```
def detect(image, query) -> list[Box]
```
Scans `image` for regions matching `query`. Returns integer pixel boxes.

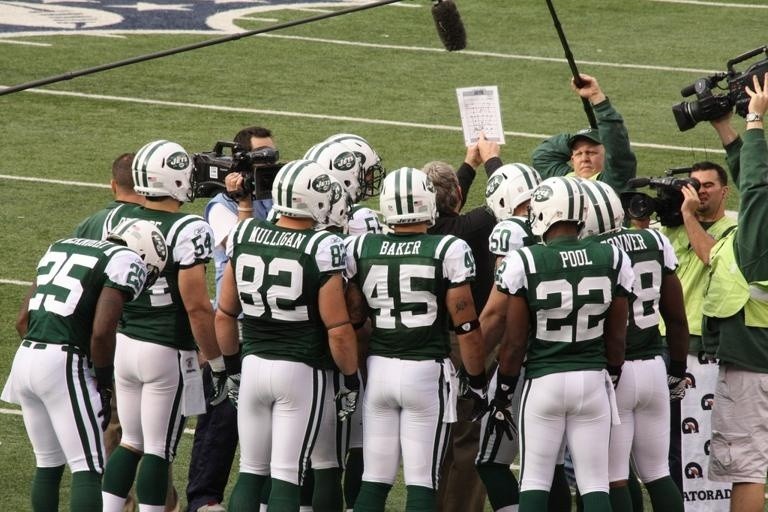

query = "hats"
[568,128,601,146]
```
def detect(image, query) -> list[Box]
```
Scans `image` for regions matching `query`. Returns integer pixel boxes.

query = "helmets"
[106,218,168,289]
[486,163,625,238]
[269,134,437,230]
[132,140,193,201]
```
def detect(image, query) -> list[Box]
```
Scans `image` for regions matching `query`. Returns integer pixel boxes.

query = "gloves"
[98,385,112,431]
[465,370,518,440]
[210,371,241,409]
[336,374,360,421]
[668,361,687,401]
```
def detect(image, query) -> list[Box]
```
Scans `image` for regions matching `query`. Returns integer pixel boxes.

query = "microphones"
[432,0,466,52]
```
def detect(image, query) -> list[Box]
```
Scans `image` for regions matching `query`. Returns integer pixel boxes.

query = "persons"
[0,72,768,512]
